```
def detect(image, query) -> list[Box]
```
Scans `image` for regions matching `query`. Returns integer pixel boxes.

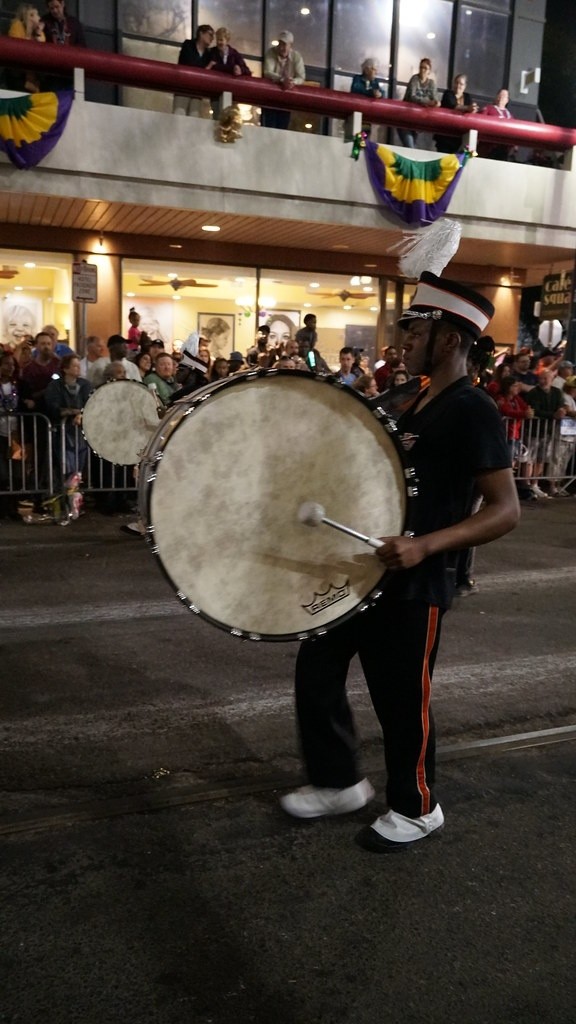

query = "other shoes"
[454,580,479,598]
[121,522,142,536]
[279,778,376,818]
[531,485,548,498]
[356,802,445,852]
[550,485,570,497]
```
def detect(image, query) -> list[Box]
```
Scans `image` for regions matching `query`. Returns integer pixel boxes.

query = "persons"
[174,25,251,120]
[467,335,576,500]
[264,314,298,347]
[433,74,479,153]
[284,220,521,842]
[0,310,332,533]
[336,345,408,399]
[0,0,85,93]
[200,317,231,360]
[397,59,440,148]
[261,31,305,129]
[351,60,385,140]
[477,89,514,161]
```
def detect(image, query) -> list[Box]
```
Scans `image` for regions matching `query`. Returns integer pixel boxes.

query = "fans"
[139,279,218,290]
[311,288,377,300]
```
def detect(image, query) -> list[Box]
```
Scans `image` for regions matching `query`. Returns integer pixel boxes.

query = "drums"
[138,366,422,642]
[78,380,167,469]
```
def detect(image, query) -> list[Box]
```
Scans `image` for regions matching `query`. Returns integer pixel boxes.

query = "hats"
[559,360,576,368]
[108,335,132,347]
[227,352,244,365]
[353,346,364,353]
[151,339,164,348]
[258,325,270,334]
[175,349,209,375]
[385,217,495,339]
